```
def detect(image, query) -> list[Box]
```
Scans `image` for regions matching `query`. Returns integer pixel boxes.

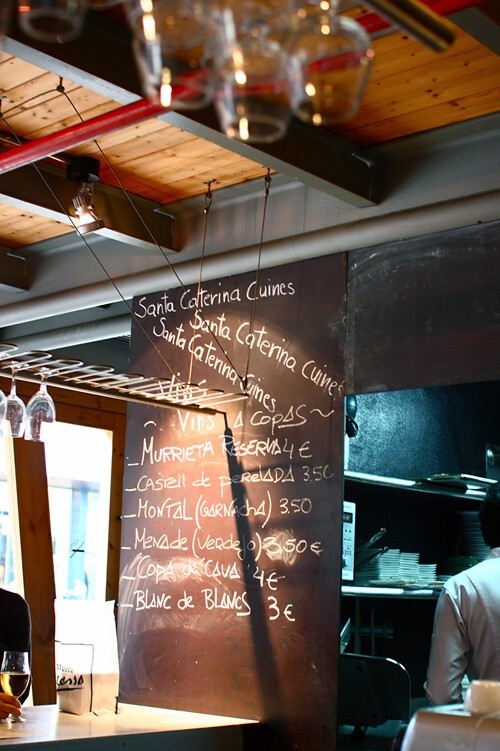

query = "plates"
[354,548,438,587]
[408,474,498,491]
[455,511,491,557]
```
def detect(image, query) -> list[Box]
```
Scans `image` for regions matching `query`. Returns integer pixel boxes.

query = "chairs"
[338,653,411,751]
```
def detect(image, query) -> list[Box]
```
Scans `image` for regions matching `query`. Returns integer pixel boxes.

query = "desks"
[0,702,259,751]
[401,703,500,751]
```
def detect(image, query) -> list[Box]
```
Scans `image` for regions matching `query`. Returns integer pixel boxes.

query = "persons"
[0,588,31,723]
[423,480,500,708]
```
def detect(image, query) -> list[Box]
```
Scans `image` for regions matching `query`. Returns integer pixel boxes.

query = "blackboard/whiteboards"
[119,252,346,751]
[345,220,500,397]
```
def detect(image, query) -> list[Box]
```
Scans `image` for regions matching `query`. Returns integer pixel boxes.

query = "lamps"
[66,156,105,236]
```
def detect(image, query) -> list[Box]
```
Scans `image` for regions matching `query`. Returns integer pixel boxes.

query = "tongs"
[354,527,389,573]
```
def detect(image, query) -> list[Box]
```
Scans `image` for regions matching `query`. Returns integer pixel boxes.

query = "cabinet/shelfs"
[340,470,484,652]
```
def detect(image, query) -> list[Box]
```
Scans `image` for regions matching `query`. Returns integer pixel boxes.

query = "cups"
[465,679,500,713]
[17,0,371,140]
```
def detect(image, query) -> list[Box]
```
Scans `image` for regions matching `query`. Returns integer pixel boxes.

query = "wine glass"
[0,650,31,724]
